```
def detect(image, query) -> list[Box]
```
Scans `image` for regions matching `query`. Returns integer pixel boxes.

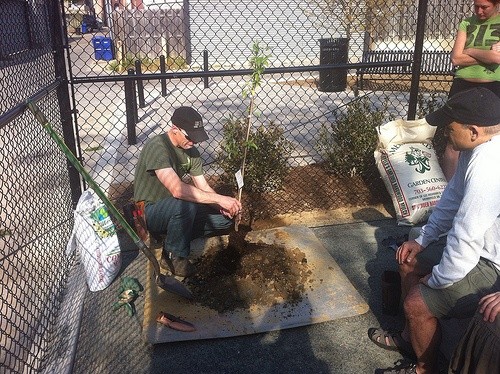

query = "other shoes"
[160,249,193,277]
[156,233,166,245]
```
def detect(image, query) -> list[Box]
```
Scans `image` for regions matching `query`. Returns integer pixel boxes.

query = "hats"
[171,106,209,144]
[425,87,500,127]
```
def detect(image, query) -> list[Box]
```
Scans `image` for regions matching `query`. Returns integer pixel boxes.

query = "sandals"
[376,363,417,374]
[368,328,415,352]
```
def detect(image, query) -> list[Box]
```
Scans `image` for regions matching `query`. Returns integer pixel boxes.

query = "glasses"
[179,128,191,140]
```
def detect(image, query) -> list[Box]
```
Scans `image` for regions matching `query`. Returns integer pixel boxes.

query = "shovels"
[24,98,195,302]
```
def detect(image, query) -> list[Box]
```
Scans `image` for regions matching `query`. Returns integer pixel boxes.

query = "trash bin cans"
[319,38,349,93]
[75,28,81,35]
[82,23,87,34]
[92,36,114,62]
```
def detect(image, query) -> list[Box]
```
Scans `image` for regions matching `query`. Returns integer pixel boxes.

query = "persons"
[446,290,500,374]
[369,88,499,374]
[133,107,242,277]
[440,0,500,181]
